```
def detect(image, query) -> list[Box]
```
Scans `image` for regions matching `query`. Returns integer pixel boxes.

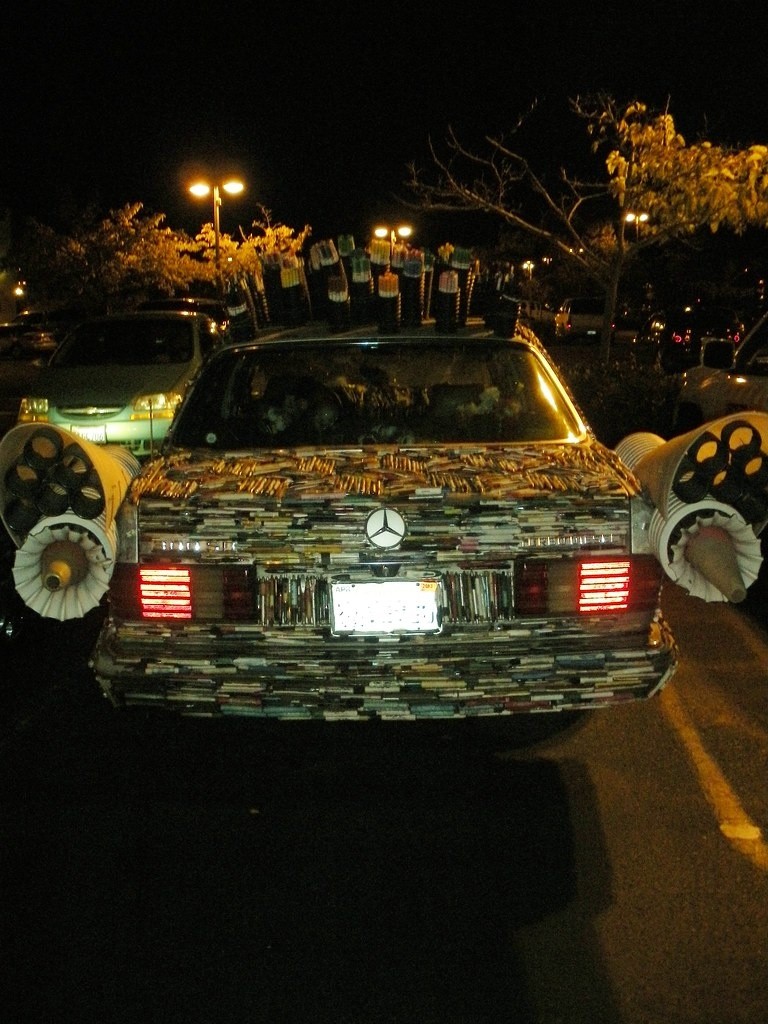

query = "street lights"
[374,226,413,270]
[522,261,535,281]
[189,176,244,298]
[627,213,649,240]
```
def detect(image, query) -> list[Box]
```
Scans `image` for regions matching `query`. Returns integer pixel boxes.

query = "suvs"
[554,295,614,344]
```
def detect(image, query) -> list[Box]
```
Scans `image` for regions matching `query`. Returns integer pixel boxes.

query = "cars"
[0,236,768,744]
[133,299,254,343]
[669,310,768,441]
[611,317,641,345]
[0,310,47,358]
[19,310,226,458]
[638,309,746,373]
[21,311,85,352]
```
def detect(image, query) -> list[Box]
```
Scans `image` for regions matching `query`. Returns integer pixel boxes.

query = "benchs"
[243,388,494,445]
